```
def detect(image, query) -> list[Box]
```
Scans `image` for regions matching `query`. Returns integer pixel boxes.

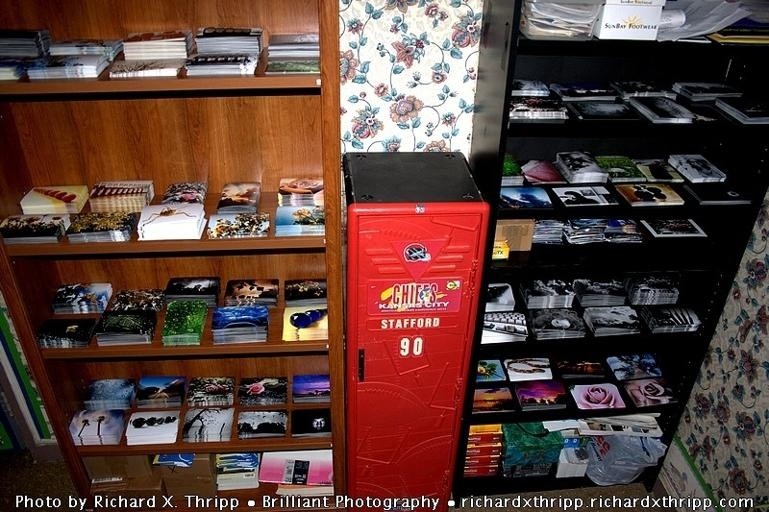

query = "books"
[0,178,325,243]
[215,450,334,497]
[498,154,752,208]
[508,79,769,125]
[480,274,701,344]
[578,417,664,437]
[69,376,331,446]
[464,423,503,477]
[519,0,769,42]
[0,27,320,80]
[36,276,328,348]
[532,217,709,243]
[472,355,678,414]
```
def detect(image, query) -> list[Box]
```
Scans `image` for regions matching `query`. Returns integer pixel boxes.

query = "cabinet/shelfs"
[1,0,347,512]
[451,0,769,510]
